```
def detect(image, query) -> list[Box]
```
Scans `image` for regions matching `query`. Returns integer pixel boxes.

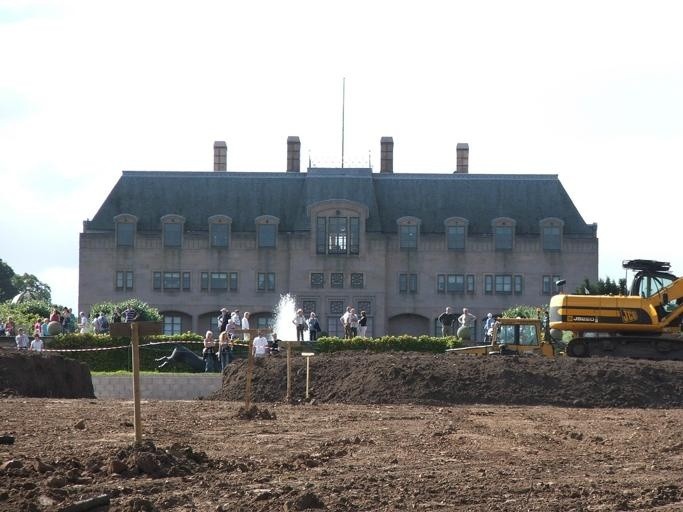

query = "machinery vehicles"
[443,317,566,362]
[548,275,682,361]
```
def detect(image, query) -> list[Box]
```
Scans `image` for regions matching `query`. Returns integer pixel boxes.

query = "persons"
[204,308,281,373]
[0,302,139,351]
[438,306,456,337]
[340,305,368,338]
[292,308,305,341]
[458,308,477,327]
[483,312,493,342]
[308,312,318,341]
